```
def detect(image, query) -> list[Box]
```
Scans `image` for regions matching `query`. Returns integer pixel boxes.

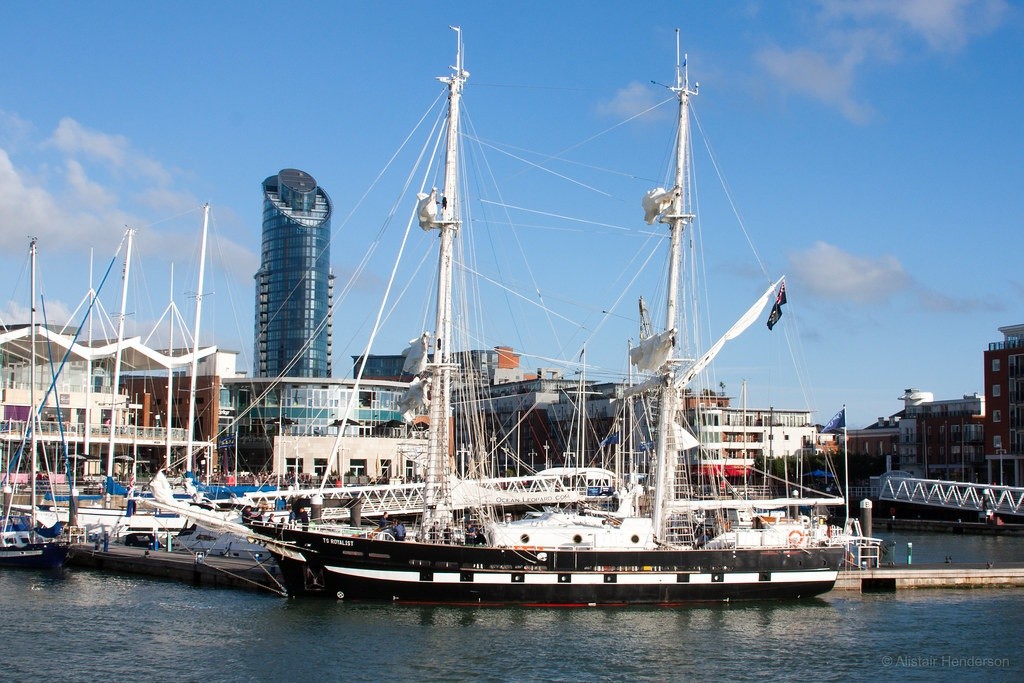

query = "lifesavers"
[788,530,803,546]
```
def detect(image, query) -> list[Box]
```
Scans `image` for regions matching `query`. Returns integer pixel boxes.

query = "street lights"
[528,449,537,469]
[456,442,470,479]
[562,445,575,467]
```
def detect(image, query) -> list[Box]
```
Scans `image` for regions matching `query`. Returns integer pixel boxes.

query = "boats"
[868,471,1024,533]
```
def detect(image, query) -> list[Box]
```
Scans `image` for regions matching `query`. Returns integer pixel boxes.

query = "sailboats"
[0,25,884,608]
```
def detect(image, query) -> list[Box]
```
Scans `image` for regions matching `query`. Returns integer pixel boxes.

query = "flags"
[766,281,788,331]
[818,409,845,433]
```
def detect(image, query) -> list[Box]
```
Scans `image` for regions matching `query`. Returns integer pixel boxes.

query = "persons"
[277,517,289,530]
[288,509,296,529]
[44,473,50,486]
[428,521,443,544]
[392,520,406,541]
[406,474,424,483]
[35,471,43,485]
[265,513,276,528]
[371,476,389,484]
[256,511,266,526]
[47,414,54,421]
[316,471,342,489]
[464,522,477,546]
[242,505,253,525]
[379,512,392,540]
[474,529,487,546]
[292,507,309,532]
[200,471,313,489]
[443,523,454,545]
